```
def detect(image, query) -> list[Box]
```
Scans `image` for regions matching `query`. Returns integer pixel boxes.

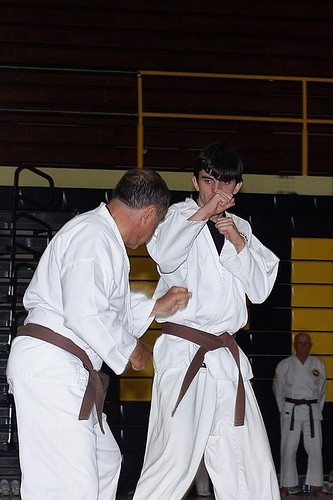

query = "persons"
[6,169,193,500]
[131,146,282,500]
[273,333,328,498]
[196,454,214,500]
